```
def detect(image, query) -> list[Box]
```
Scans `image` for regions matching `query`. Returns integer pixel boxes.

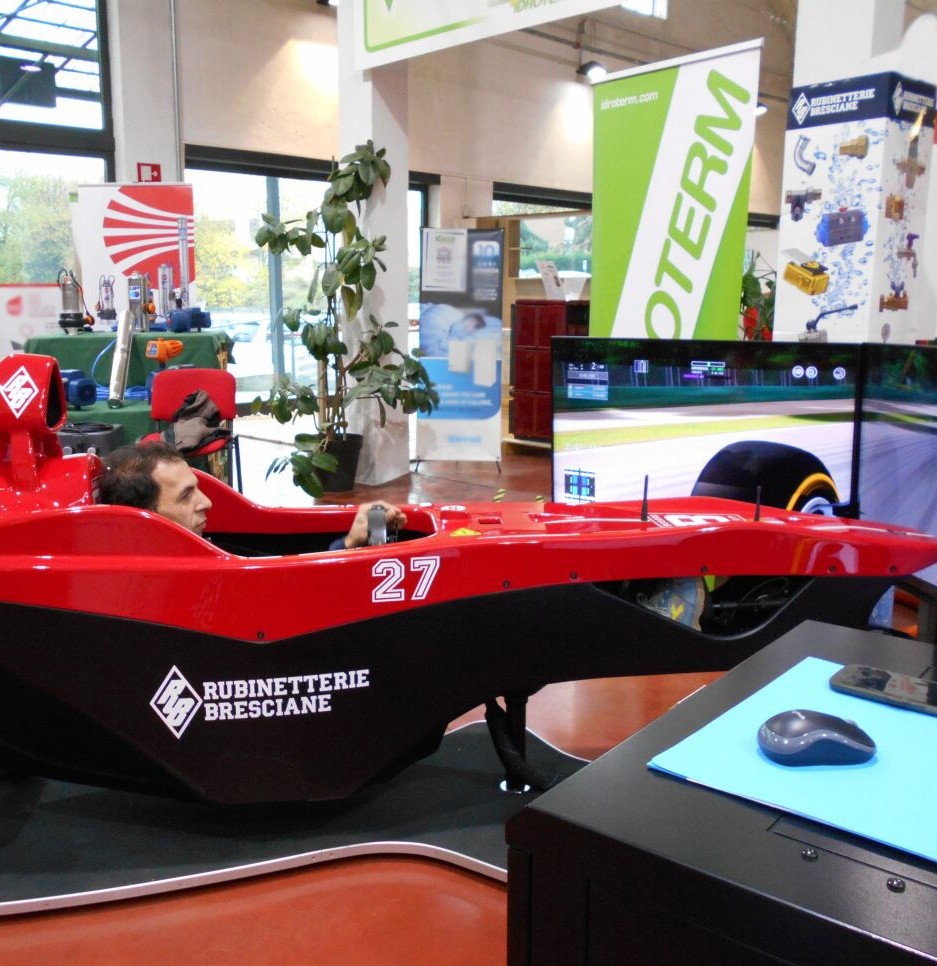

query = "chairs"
[150,367,243,494]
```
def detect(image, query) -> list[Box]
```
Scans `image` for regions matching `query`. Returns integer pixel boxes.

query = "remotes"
[831,664,936,714]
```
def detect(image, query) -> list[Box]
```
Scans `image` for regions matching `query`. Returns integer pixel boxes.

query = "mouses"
[759,710,877,764]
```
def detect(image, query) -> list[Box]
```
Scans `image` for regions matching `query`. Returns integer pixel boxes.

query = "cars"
[230,316,314,354]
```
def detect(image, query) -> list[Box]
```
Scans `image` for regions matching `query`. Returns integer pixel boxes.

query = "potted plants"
[255,140,440,497]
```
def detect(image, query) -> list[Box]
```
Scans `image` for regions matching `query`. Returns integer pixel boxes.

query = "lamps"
[577,60,605,79]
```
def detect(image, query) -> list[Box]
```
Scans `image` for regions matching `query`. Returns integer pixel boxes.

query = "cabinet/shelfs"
[507,299,590,438]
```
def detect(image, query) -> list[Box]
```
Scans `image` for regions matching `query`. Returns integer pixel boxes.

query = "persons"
[96,440,408,551]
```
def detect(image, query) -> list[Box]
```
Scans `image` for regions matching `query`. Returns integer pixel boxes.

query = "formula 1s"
[2,352,937,811]
